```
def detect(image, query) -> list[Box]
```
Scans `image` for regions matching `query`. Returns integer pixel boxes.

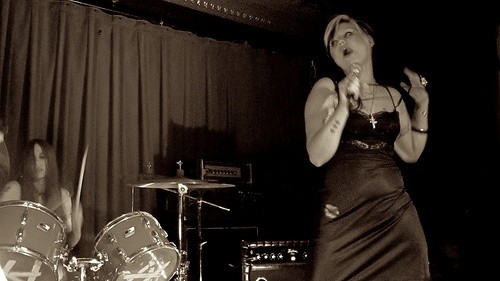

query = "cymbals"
[125,178,236,190]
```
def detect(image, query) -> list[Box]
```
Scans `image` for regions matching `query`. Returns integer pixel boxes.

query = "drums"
[0,200,70,281]
[67,256,96,280]
[90,210,182,281]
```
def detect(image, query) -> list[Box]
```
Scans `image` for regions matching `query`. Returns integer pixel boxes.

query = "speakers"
[157,189,316,281]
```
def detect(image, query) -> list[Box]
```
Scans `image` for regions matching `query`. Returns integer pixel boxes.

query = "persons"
[305,14,430,281]
[0,118,84,281]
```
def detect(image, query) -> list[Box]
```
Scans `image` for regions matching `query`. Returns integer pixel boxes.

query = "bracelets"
[411,126,429,134]
[414,108,428,118]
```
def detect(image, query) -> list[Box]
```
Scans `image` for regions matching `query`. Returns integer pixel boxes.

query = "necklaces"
[38,191,43,196]
[363,81,377,128]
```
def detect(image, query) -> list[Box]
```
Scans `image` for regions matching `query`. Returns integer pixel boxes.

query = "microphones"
[349,63,361,111]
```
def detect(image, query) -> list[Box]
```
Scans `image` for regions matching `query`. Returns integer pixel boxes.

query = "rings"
[419,75,428,86]
[407,86,411,94]
